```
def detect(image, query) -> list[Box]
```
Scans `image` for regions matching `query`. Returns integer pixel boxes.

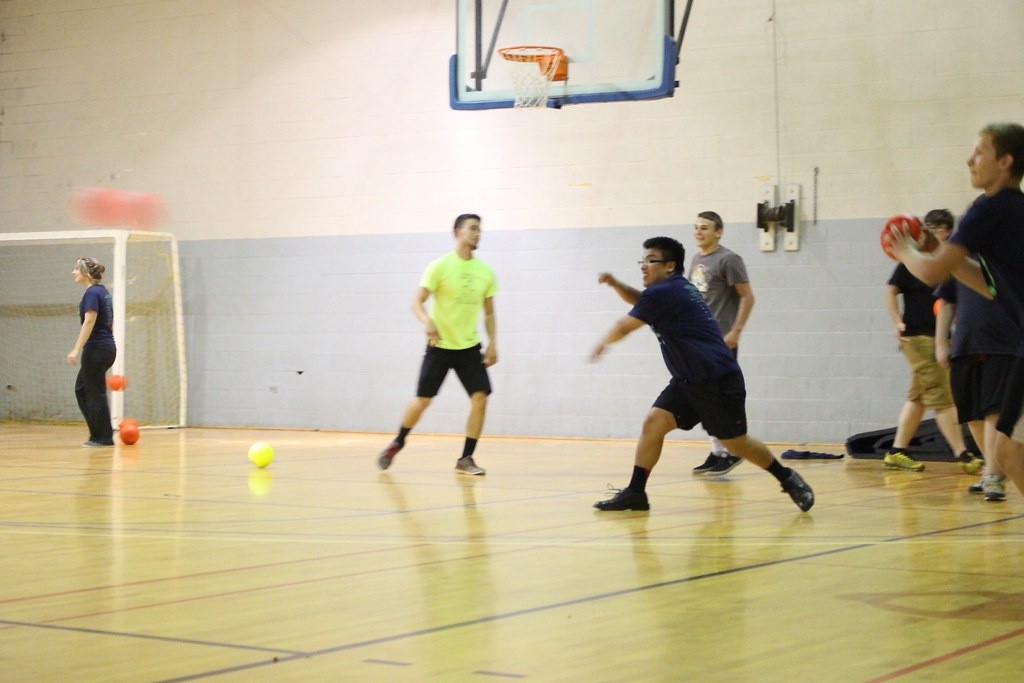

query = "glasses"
[638,260,675,267]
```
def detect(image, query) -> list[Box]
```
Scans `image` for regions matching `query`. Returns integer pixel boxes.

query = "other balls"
[880,215,931,263]
[119,418,139,430]
[248,442,274,468]
[120,425,140,445]
[106,374,122,391]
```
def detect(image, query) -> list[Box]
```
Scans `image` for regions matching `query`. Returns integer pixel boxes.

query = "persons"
[881,122,1024,500]
[685,210,754,477]
[589,237,816,513]
[378,213,499,477]
[68,258,117,447]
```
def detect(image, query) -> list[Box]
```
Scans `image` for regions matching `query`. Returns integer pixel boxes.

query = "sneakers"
[957,452,985,474]
[379,436,404,469]
[710,455,744,476]
[983,473,1007,500]
[881,450,925,472]
[456,454,485,476]
[692,451,723,473]
[969,479,984,494]
[593,484,649,510]
[780,467,814,511]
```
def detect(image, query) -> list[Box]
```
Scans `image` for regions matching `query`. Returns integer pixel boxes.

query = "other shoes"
[83,441,116,448]
